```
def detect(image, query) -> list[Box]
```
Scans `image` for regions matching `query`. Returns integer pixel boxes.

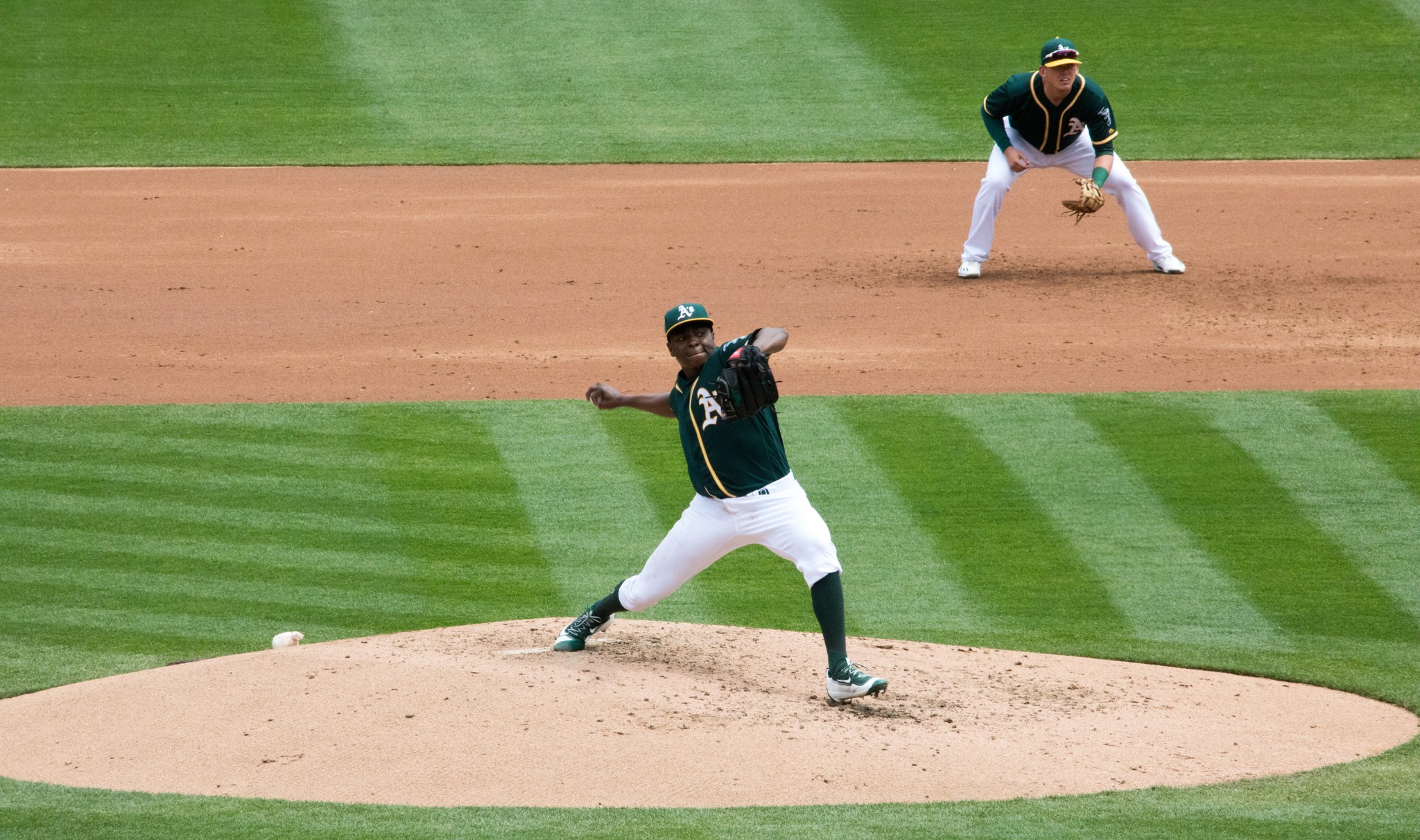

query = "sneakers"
[1153,254,1186,272]
[959,260,981,278]
[826,656,889,704]
[554,604,615,652]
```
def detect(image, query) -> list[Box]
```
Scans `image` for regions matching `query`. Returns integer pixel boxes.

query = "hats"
[1040,36,1083,67]
[664,303,714,339]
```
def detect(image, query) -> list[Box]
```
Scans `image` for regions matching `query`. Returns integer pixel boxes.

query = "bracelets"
[1092,167,1110,189]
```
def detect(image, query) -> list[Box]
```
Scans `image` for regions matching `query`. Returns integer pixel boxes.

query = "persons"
[554,304,888,703]
[957,36,1186,276]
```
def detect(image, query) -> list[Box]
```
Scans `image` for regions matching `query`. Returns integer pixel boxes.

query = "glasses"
[1042,50,1080,64]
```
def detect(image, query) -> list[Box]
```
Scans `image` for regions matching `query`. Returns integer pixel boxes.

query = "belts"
[759,488,768,494]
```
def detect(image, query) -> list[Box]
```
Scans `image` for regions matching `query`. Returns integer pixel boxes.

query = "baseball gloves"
[1062,178,1105,214]
[717,345,778,424]
